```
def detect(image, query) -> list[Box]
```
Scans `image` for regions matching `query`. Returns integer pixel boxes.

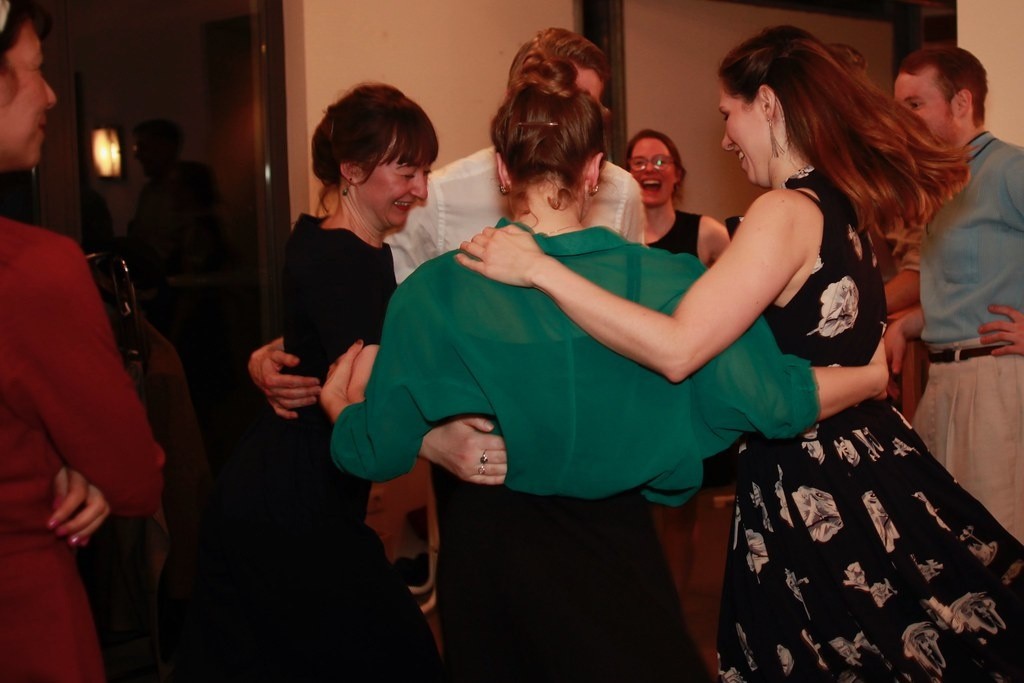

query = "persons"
[246,27,649,683]
[318,54,894,683]
[0,0,167,683]
[187,83,508,683]
[819,40,923,314]
[625,128,732,608]
[884,45,1024,546]
[68,101,251,683]
[454,24,1024,683]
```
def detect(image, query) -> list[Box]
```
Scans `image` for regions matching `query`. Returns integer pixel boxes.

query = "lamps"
[91,127,127,183]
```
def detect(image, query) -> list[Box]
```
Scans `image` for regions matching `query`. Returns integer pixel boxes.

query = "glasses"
[628,154,673,172]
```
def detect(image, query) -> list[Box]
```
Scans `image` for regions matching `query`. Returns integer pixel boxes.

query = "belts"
[928,344,1005,363]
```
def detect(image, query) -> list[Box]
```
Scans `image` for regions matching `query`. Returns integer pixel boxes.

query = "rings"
[480,449,489,464]
[478,464,488,475]
